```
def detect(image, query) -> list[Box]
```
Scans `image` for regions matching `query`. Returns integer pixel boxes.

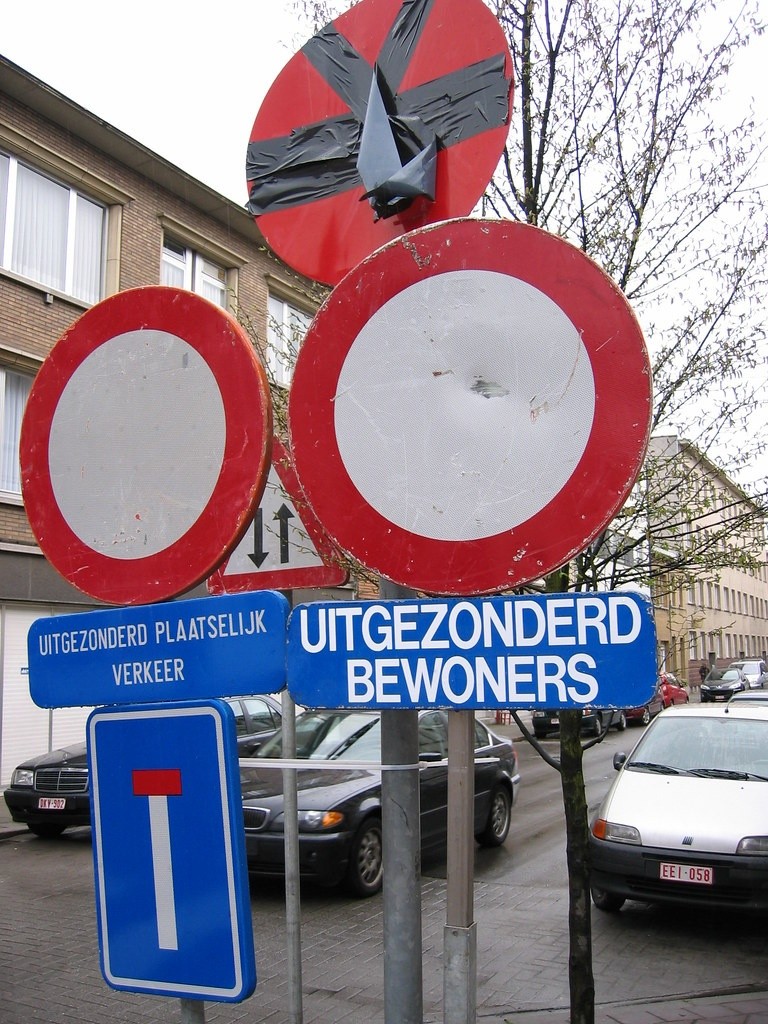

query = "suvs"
[727,658,768,691]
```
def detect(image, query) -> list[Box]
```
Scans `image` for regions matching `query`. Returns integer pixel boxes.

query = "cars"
[4,695,283,837]
[532,708,627,740]
[236,708,520,898]
[727,690,768,703]
[624,687,666,726]
[700,668,751,703]
[659,672,690,708]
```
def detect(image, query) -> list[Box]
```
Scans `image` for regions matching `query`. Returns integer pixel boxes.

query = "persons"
[699,662,710,683]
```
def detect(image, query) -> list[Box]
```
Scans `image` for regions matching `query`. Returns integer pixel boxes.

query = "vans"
[586,700,768,919]
